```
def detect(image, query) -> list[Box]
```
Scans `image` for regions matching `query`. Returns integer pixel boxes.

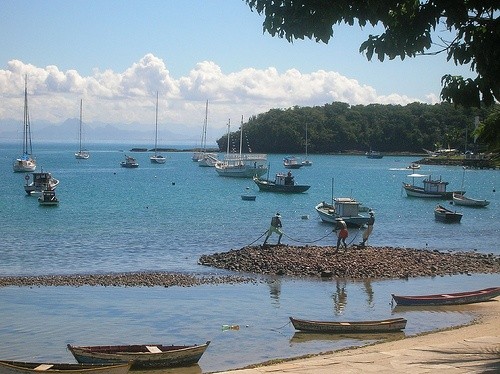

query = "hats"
[368,208,375,213]
[275,212,282,218]
[334,215,344,221]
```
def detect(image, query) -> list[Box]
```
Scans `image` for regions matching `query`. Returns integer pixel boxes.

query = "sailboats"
[75,99,90,159]
[150,89,167,164]
[13,74,36,172]
[191,99,268,178]
[452,126,490,208]
[302,123,312,165]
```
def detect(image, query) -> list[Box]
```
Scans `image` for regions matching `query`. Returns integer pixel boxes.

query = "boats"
[253,161,311,194]
[119,154,139,168]
[433,202,463,223]
[288,316,407,332]
[0,359,135,374]
[314,178,375,228]
[23,165,59,205]
[65,339,211,368]
[283,155,303,168]
[406,163,421,169]
[364,146,383,159]
[401,174,467,199]
[391,287,500,307]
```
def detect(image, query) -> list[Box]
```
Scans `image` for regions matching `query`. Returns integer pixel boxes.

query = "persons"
[264,213,283,245]
[359,211,374,245]
[333,216,347,252]
